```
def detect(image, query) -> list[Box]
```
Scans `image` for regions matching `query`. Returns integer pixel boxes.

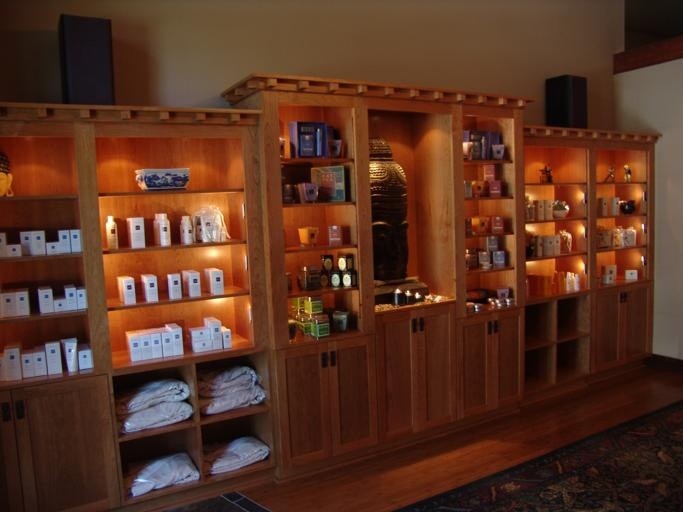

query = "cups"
[303,182,319,202]
[327,139,342,157]
[492,143,505,159]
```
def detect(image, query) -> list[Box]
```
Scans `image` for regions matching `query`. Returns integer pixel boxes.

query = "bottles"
[550,270,579,294]
[152,212,193,248]
[103,214,119,251]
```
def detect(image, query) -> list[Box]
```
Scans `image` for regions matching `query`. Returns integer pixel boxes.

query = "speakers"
[544,75,588,127]
[58,13,115,105]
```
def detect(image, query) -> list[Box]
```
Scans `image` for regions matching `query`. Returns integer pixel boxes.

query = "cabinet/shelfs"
[221,73,537,483]
[0,106,275,511]
[520,124,659,416]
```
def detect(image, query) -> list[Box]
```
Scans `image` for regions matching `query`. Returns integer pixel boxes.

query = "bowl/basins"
[552,209,569,219]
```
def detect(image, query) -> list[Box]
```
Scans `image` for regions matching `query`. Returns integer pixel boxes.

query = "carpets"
[397,396,683,512]
